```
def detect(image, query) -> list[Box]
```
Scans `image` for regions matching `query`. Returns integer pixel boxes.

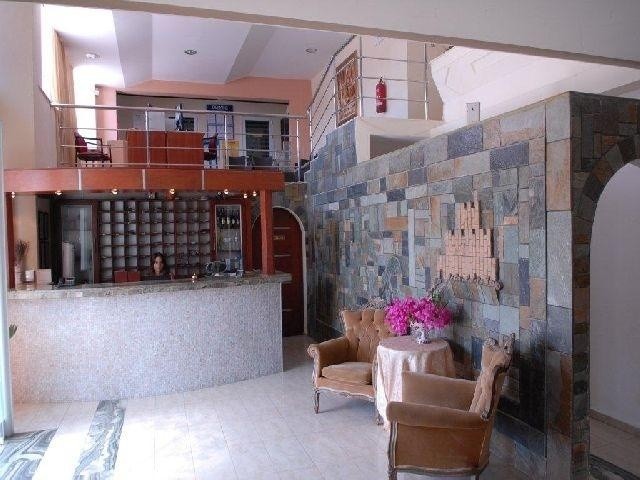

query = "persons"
[143,251,177,281]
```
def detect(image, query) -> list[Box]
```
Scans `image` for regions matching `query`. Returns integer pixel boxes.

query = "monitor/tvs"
[174,103,184,131]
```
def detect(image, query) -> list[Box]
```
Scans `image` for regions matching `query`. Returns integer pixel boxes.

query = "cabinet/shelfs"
[97,200,254,282]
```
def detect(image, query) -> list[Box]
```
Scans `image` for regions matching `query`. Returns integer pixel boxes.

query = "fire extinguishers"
[376,75,386,113]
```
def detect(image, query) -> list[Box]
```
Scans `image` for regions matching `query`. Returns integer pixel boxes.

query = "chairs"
[225,153,281,172]
[386,333,517,480]
[74,133,113,167]
[307,308,395,412]
[204,132,219,168]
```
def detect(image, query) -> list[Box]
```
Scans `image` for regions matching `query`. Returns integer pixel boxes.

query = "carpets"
[0,399,128,480]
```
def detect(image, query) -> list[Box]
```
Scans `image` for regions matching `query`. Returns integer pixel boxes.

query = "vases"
[410,320,432,343]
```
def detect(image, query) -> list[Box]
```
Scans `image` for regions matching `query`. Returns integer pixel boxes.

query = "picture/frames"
[333,49,362,127]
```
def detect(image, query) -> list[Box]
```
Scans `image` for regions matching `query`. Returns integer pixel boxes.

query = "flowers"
[385,277,453,335]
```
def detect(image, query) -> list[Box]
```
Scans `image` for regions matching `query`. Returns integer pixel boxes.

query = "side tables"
[373,334,454,431]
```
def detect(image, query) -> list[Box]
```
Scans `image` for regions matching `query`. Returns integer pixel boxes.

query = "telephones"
[59,276,76,287]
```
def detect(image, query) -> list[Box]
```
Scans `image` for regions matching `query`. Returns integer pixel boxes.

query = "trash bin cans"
[110,140,129,167]
[220,140,239,170]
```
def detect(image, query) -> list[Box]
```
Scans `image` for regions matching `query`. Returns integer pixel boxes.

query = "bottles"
[219,208,240,229]
